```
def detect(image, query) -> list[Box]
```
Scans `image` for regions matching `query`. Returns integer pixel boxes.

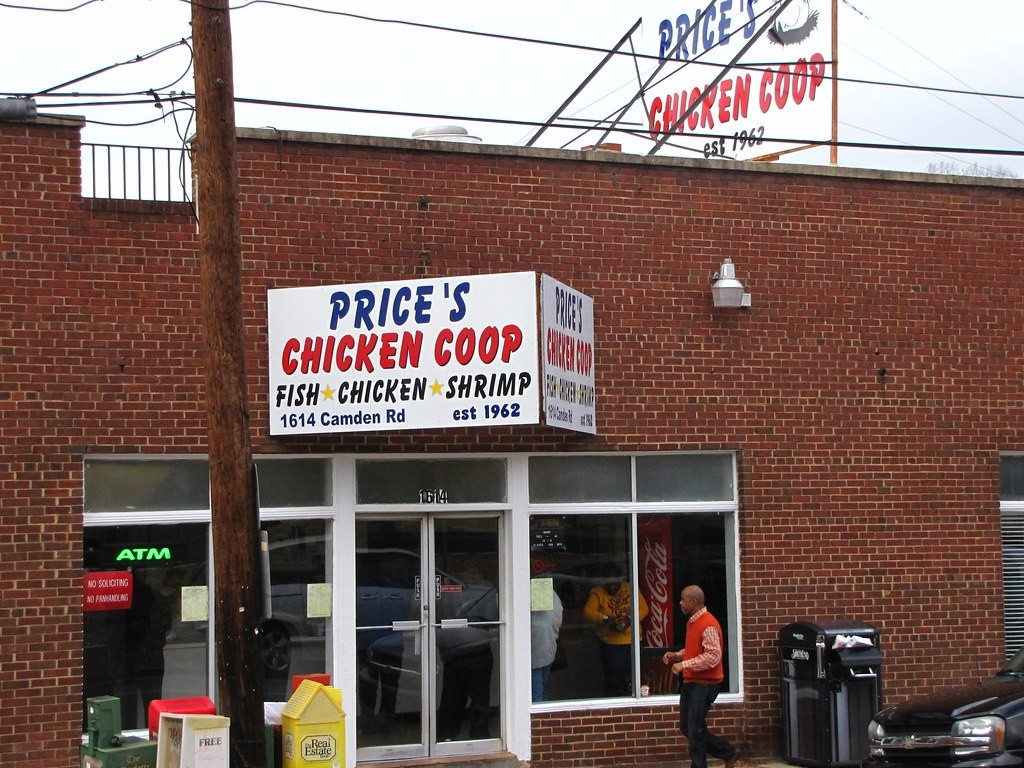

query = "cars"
[860,641,1024,768]
[158,532,615,732]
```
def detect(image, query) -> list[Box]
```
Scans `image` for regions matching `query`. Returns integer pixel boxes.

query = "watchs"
[603,614,609,623]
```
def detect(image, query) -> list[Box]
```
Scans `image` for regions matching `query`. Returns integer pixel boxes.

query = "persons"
[582,563,649,697]
[356,552,413,734]
[663,585,741,768]
[530,588,563,702]
[436,617,494,743]
[122,561,173,730]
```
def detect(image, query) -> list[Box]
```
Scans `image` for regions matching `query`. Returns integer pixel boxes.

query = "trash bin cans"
[777,619,884,768]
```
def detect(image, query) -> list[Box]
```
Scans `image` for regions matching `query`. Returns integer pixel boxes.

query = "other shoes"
[725,748,741,768]
